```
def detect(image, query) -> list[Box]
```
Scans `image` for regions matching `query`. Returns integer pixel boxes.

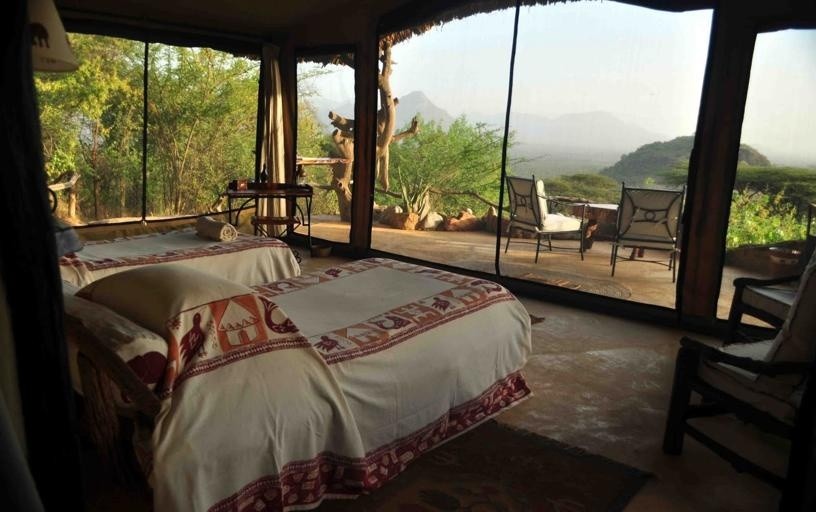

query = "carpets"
[306,417,658,512]
[529,312,544,324]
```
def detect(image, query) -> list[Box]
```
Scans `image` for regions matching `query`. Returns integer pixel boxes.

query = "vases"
[260,164,269,183]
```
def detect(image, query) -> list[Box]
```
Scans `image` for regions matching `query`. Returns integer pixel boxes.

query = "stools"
[251,215,302,263]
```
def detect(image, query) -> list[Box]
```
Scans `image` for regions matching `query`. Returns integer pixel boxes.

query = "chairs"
[663,250,815,512]
[503,171,586,264]
[608,181,686,283]
[722,233,816,347]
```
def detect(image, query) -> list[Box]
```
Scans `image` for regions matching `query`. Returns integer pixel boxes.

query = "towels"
[194,216,238,241]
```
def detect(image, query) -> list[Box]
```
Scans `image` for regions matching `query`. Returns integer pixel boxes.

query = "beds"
[51,226,301,288]
[59,258,535,512]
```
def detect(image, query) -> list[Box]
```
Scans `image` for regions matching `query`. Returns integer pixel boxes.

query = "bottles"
[294,165,308,187]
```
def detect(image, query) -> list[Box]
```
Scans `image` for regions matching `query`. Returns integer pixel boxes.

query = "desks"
[224,183,314,249]
[568,201,645,259]
